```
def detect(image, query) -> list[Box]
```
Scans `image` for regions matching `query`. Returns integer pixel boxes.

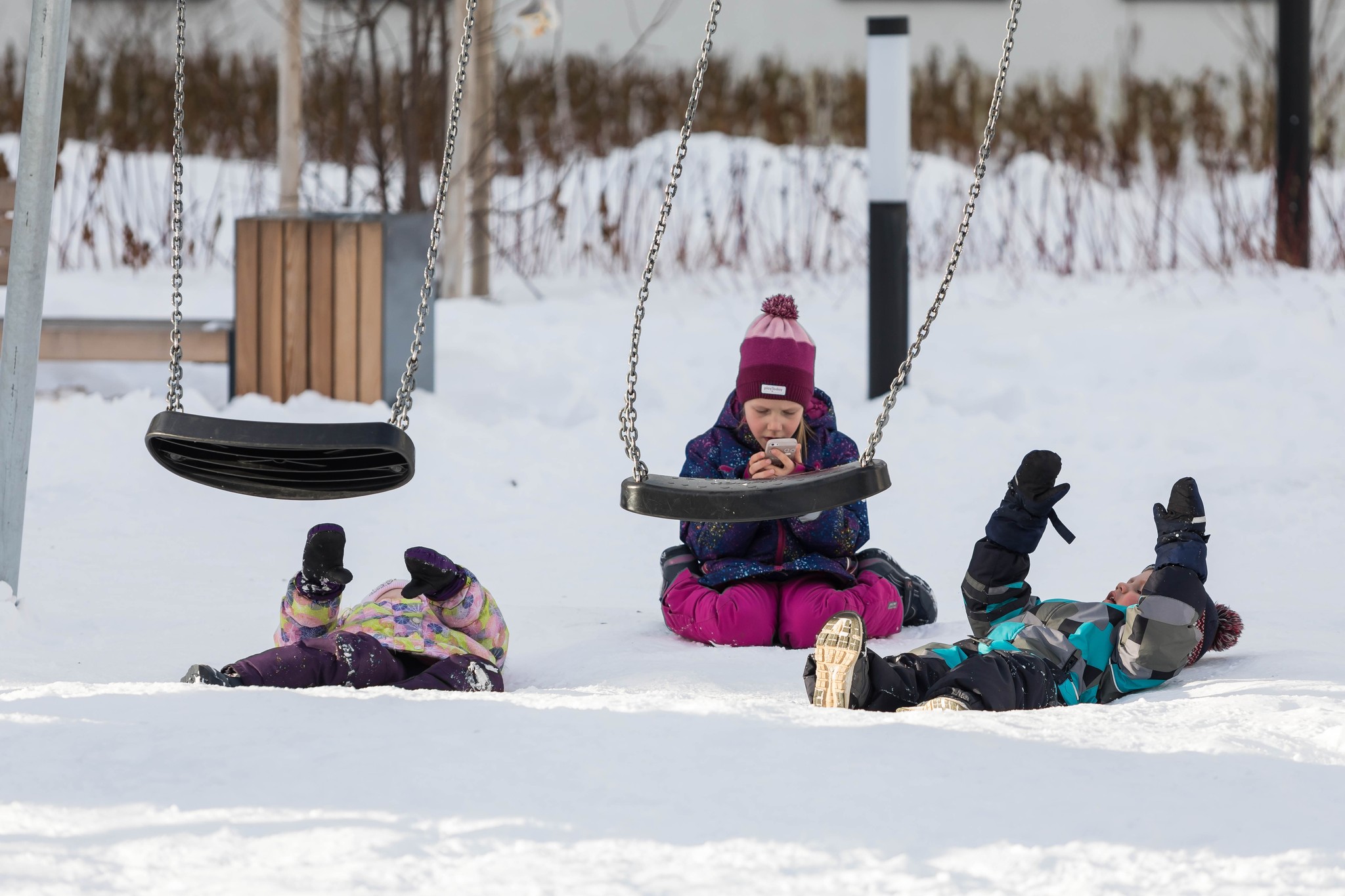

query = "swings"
[141,0,477,504]
[609,0,1023,526]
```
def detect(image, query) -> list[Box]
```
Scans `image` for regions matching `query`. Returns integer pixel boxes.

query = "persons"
[660,294,938,651]
[803,449,1243,713]
[179,523,509,693]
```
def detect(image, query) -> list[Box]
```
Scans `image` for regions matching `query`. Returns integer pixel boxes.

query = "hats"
[1143,564,1244,667]
[736,294,817,408]
[367,578,426,607]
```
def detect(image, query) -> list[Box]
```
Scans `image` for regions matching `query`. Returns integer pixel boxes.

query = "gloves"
[1152,476,1214,542]
[295,523,354,602]
[985,449,1076,553]
[401,546,467,601]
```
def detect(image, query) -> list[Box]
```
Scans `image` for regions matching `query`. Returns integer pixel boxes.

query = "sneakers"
[896,695,972,712]
[660,544,697,601]
[851,547,936,627]
[814,610,870,710]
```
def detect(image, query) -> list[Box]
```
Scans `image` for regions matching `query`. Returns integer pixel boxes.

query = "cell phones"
[763,438,797,469]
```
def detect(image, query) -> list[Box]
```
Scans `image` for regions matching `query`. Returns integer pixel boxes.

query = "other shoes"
[179,664,230,687]
